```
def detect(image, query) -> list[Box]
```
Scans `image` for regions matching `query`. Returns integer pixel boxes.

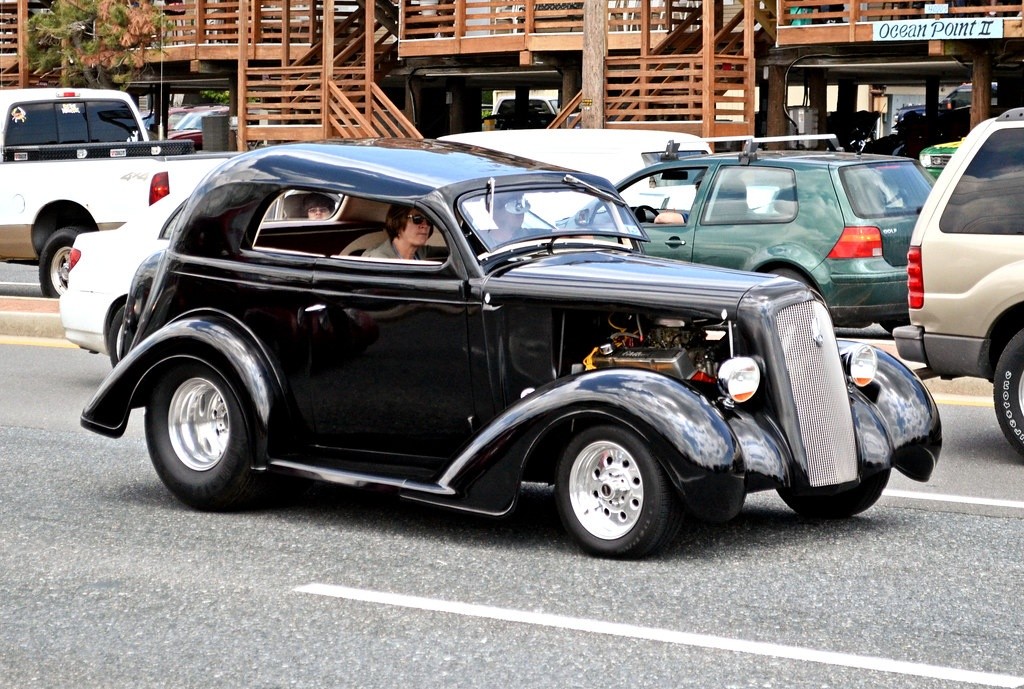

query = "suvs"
[891,106,1024,464]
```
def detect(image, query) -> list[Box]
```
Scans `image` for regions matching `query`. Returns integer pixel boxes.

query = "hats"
[692,169,705,185]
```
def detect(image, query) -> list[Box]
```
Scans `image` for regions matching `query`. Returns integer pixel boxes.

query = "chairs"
[709,177,754,221]
[493,0,699,35]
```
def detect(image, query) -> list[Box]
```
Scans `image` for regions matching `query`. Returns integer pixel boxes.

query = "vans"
[437,127,714,230]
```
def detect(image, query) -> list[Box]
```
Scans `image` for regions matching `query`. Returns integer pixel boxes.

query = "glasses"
[695,186,698,189]
[649,181,655,183]
[307,208,329,213]
[489,197,524,209]
[407,213,434,226]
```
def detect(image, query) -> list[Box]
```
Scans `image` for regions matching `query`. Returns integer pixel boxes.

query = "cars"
[553,134,937,332]
[493,98,559,130]
[893,82,998,135]
[77,137,943,560]
[58,170,556,359]
[146,106,228,146]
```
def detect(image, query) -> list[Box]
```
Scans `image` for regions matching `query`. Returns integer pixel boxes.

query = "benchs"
[340,231,446,257]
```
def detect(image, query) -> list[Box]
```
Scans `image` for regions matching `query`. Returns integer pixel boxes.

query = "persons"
[476,194,552,250]
[303,192,335,219]
[365,204,433,261]
[654,170,706,224]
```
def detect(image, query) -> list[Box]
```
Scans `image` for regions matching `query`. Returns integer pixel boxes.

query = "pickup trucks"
[1,88,244,299]
